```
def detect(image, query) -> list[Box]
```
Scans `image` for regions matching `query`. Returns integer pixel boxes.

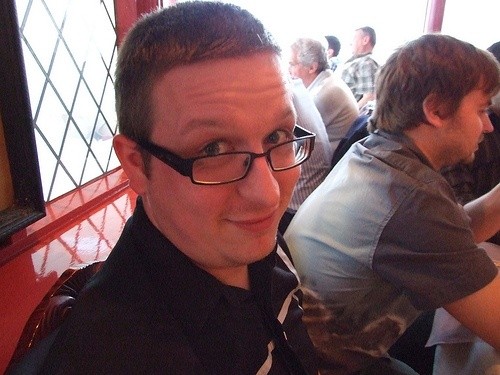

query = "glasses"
[125,124,317,186]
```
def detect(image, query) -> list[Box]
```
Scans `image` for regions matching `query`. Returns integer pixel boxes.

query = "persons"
[278,26,382,236]
[283,33,500,375]
[37,0,320,375]
